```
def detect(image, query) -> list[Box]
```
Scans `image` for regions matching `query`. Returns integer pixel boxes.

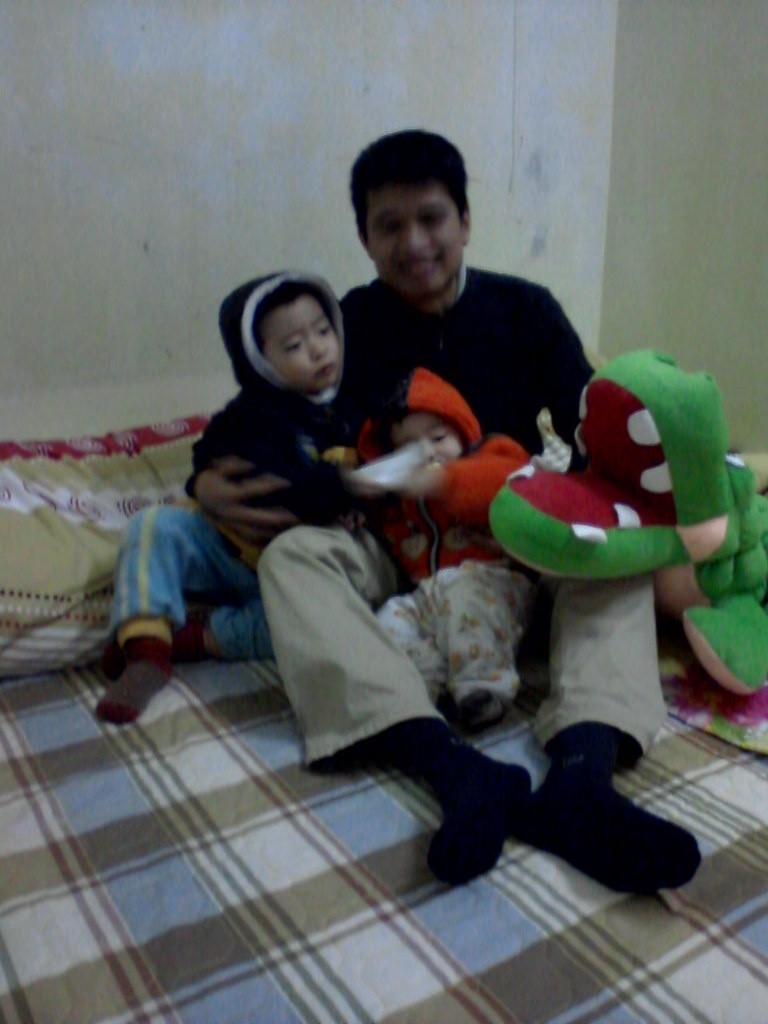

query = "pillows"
[1,411,213,680]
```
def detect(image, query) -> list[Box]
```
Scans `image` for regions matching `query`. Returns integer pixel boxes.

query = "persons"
[98,270,366,684]
[185,129,703,895]
[357,369,536,730]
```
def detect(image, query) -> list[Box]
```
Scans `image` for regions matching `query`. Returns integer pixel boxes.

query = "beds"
[0,654,767,1024]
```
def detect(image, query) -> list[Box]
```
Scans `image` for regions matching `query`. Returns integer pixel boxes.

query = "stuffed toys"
[491,351,768,694]
